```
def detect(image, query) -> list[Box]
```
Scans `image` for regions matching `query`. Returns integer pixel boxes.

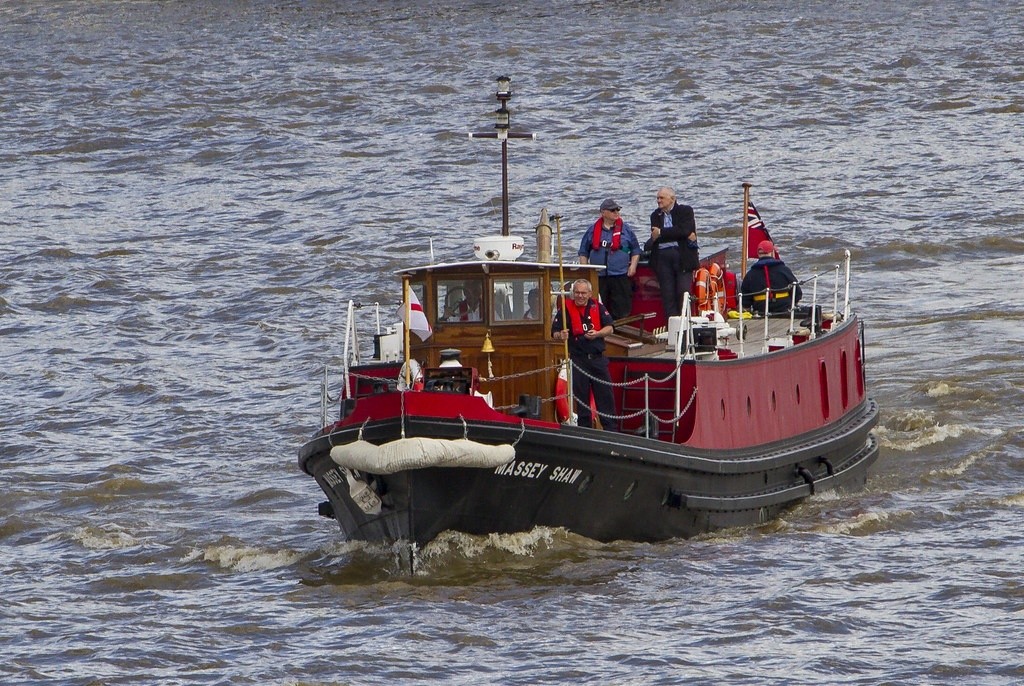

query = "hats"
[756,240,778,253]
[599,198,623,210]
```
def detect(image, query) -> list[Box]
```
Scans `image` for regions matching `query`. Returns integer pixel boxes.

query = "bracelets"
[659,229,660,235]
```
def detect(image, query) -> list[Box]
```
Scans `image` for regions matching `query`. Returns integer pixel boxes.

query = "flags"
[748,200,780,260]
[395,286,433,342]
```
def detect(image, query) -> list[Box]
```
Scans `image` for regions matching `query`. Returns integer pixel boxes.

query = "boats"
[296,78,882,577]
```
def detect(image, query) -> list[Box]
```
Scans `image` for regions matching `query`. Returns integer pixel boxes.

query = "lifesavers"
[397,359,425,393]
[555,358,579,424]
[696,263,727,316]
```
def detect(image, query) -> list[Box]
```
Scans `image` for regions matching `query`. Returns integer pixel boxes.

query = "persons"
[550,279,619,432]
[439,280,501,320]
[649,186,700,318]
[578,198,640,320]
[521,288,539,319]
[740,240,802,319]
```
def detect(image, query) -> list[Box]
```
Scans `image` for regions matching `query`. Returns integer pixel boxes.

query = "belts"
[576,352,603,359]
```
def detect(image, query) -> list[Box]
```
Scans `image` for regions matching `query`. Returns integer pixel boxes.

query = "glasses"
[609,207,620,212]
